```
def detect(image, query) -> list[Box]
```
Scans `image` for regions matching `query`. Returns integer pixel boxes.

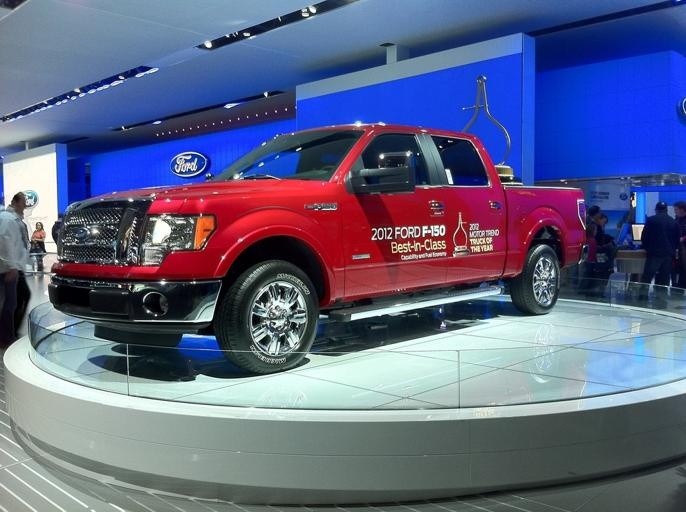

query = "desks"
[616,249,647,291]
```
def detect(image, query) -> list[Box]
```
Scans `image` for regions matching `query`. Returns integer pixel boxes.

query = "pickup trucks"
[46,121,591,375]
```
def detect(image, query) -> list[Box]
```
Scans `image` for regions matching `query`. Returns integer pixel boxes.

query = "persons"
[0,192,32,350]
[30,222,46,280]
[671,200,686,295]
[634,202,674,305]
[570,203,616,302]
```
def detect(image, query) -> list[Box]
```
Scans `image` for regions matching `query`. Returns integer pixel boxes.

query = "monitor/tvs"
[618,223,629,242]
[631,223,645,241]
